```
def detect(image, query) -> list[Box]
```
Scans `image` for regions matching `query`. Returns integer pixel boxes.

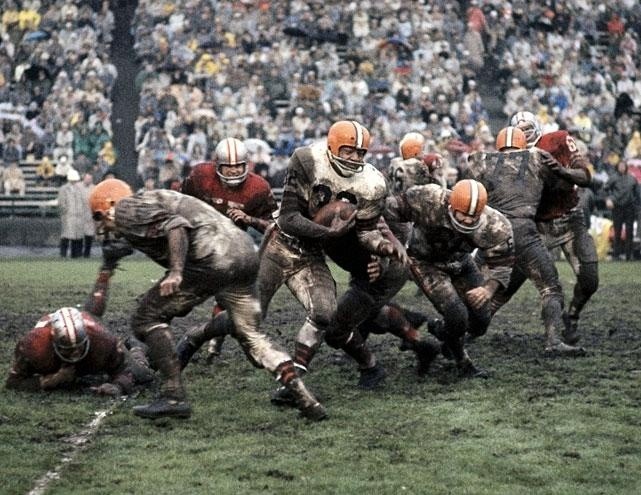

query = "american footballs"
[314,201,356,227]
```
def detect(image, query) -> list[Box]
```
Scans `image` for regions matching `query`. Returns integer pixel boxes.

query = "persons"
[90,178,329,421]
[323,228,441,388]
[384,177,515,379]
[460,126,586,355]
[177,120,390,405]
[6,243,152,396]
[180,138,278,365]
[491,110,599,344]
[0,0,641,265]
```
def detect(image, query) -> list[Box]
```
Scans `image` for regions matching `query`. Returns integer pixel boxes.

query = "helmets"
[398,129,424,160]
[447,178,488,233]
[214,138,249,187]
[422,152,446,175]
[326,118,372,177]
[495,125,526,152]
[49,305,90,366]
[88,178,132,230]
[511,111,543,148]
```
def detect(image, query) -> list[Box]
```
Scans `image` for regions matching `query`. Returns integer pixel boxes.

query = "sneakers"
[126,335,149,356]
[544,340,586,361]
[560,328,581,347]
[298,403,328,422]
[396,310,490,381]
[355,362,388,390]
[132,397,194,419]
[206,352,229,366]
[270,386,297,407]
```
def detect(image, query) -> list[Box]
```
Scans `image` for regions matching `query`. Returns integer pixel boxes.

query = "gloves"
[85,270,110,318]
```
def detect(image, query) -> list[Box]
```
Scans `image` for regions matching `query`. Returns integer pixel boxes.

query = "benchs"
[0,159,69,215]
